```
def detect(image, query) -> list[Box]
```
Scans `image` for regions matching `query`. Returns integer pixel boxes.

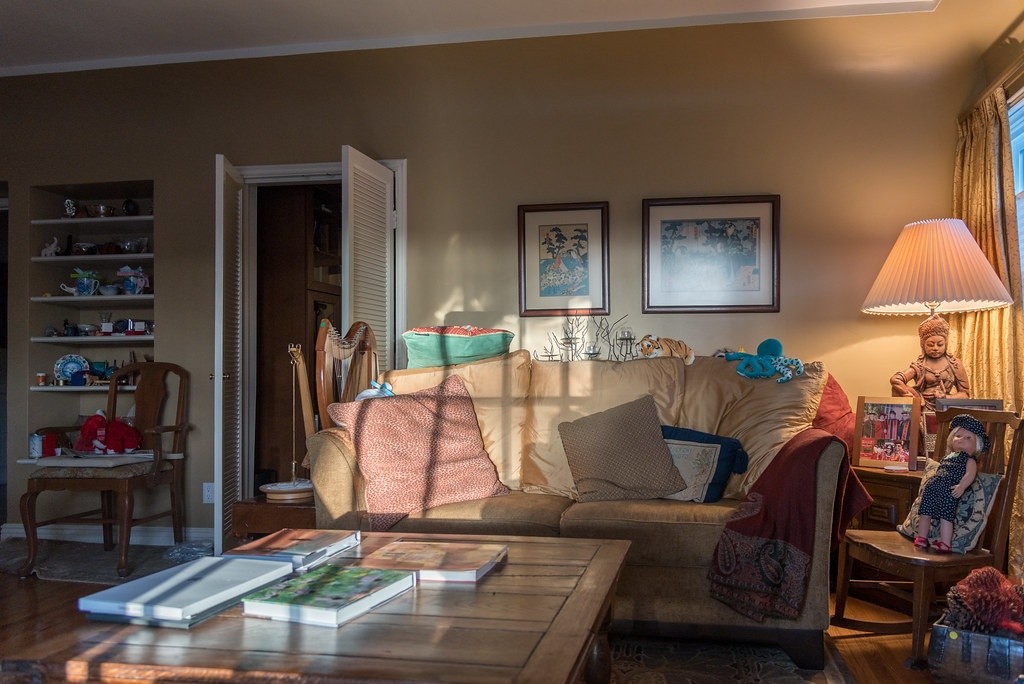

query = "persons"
[911,415,990,553]
[889,314,969,409]
[862,409,909,463]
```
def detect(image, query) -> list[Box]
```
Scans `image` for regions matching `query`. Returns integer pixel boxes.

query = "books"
[348,541,509,582]
[222,529,362,567]
[80,555,296,630]
[240,564,418,629]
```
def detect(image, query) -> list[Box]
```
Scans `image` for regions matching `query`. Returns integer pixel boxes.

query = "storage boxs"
[100,322,113,332]
[135,321,145,332]
[89,362,108,372]
[71,371,104,386]
[925,612,1024,684]
[125,330,145,336]
[255,469,278,498]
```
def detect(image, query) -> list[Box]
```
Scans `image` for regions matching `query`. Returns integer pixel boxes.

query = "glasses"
[886,448,893,451]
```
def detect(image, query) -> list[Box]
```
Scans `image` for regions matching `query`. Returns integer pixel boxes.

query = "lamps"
[857,218,1015,412]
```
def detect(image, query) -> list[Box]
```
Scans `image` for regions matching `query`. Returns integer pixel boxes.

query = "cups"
[123,276,144,295]
[139,238,148,252]
[37,373,46,386]
[76,278,99,296]
[99,312,110,323]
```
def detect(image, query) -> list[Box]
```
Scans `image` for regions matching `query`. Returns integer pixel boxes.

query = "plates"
[54,354,89,384]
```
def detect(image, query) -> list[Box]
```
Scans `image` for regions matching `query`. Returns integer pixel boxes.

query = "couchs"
[307,357,857,673]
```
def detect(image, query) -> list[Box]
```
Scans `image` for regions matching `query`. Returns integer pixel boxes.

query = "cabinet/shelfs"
[256,183,342,483]
[16,216,155,465]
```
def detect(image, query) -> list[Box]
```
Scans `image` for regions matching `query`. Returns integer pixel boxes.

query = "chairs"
[829,405,1024,674]
[18,362,191,578]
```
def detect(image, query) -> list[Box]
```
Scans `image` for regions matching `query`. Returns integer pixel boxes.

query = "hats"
[894,440,904,445]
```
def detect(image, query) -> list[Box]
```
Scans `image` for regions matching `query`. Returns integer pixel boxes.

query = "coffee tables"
[0,526,632,684]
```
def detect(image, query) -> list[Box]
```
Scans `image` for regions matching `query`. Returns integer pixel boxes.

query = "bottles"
[29,434,42,458]
[144,199,150,215]
[64,320,69,336]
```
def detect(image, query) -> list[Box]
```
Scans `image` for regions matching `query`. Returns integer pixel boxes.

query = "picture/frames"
[639,193,781,314]
[935,397,1004,412]
[851,395,921,472]
[516,201,610,318]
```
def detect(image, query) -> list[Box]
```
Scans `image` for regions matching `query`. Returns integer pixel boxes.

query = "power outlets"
[202,482,214,504]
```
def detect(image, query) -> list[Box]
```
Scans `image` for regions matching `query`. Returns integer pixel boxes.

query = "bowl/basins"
[121,240,144,254]
[85,206,115,217]
[99,286,120,295]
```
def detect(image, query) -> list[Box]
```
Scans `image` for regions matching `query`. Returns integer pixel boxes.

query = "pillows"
[895,457,1006,556]
[558,393,686,505]
[401,322,515,370]
[661,424,742,504]
[325,374,513,534]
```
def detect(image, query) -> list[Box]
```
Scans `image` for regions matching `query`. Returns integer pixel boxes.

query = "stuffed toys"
[634,335,696,365]
[81,414,141,455]
[725,338,804,384]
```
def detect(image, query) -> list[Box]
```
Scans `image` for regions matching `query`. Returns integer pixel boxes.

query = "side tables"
[849,467,923,531]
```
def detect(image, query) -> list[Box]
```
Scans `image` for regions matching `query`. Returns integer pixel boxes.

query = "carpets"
[581,632,857,684]
[0,537,214,587]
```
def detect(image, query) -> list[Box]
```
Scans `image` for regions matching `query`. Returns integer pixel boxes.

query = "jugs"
[60,283,80,296]
[122,199,137,216]
